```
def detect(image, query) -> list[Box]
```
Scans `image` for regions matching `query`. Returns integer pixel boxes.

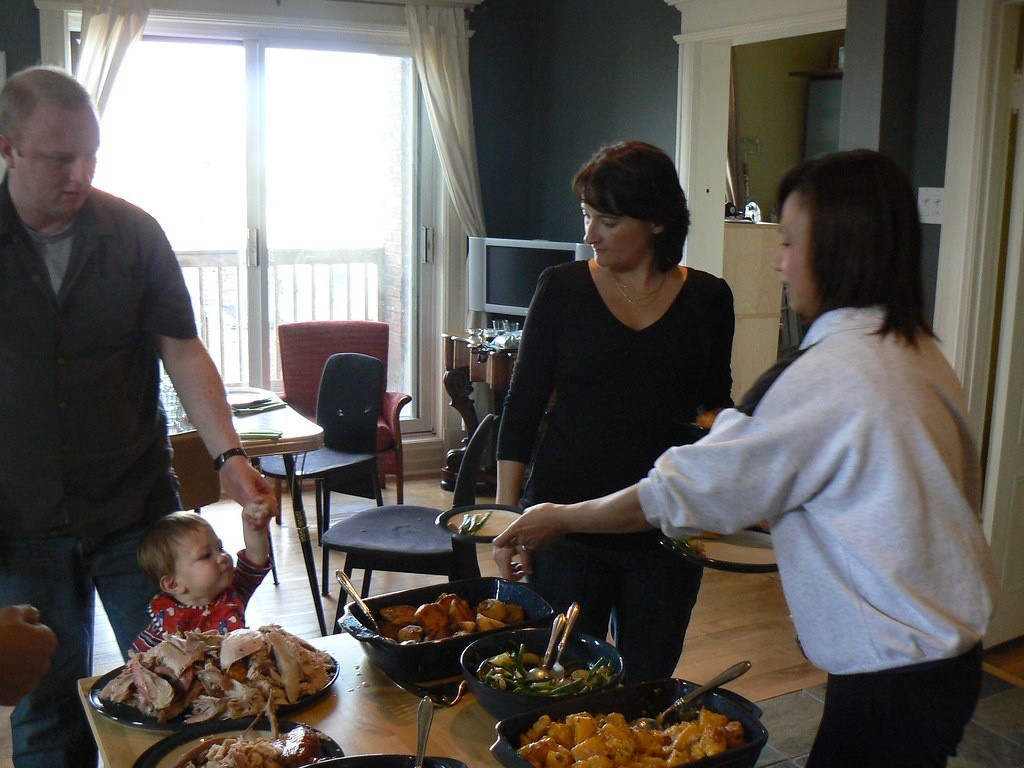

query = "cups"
[468,319,519,342]
[159,375,183,427]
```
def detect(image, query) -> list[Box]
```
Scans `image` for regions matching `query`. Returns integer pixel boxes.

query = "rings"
[522,545,530,553]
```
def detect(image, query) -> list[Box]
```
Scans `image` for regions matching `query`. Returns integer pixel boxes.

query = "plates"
[88,655,339,730]
[132,718,345,768]
[659,526,779,573]
[226,387,274,408]
[435,504,524,544]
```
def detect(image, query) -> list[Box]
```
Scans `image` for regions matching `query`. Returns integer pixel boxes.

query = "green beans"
[476,643,619,696]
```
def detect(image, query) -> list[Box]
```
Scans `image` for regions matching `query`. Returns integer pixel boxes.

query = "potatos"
[694,412,716,428]
[516,708,747,768]
[375,593,524,643]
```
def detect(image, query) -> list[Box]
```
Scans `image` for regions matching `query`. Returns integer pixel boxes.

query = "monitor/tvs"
[468,237,594,316]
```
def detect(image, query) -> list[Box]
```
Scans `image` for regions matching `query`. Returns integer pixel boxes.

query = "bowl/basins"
[460,627,625,720]
[301,754,469,768]
[337,577,555,666]
[489,678,768,768]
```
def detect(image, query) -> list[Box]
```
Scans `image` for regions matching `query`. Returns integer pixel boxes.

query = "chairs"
[279,320,412,508]
[321,412,496,634]
[260,353,387,595]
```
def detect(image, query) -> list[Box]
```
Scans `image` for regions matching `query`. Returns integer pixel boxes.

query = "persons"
[492,150,1002,768]
[132,494,279,653]
[492,139,735,677]
[0,68,273,768]
[0,607,58,706]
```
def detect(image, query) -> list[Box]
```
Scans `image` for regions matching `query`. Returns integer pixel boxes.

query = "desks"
[224,387,329,638]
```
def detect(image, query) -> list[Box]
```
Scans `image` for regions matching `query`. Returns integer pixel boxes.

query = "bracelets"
[214,448,247,470]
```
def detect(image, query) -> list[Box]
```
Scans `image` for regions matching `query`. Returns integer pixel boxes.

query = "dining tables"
[78,632,501,768]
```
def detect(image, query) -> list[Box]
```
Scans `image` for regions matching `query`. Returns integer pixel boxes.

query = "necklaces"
[608,268,666,305]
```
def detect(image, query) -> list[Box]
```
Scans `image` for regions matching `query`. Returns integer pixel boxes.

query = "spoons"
[526,599,580,678]
